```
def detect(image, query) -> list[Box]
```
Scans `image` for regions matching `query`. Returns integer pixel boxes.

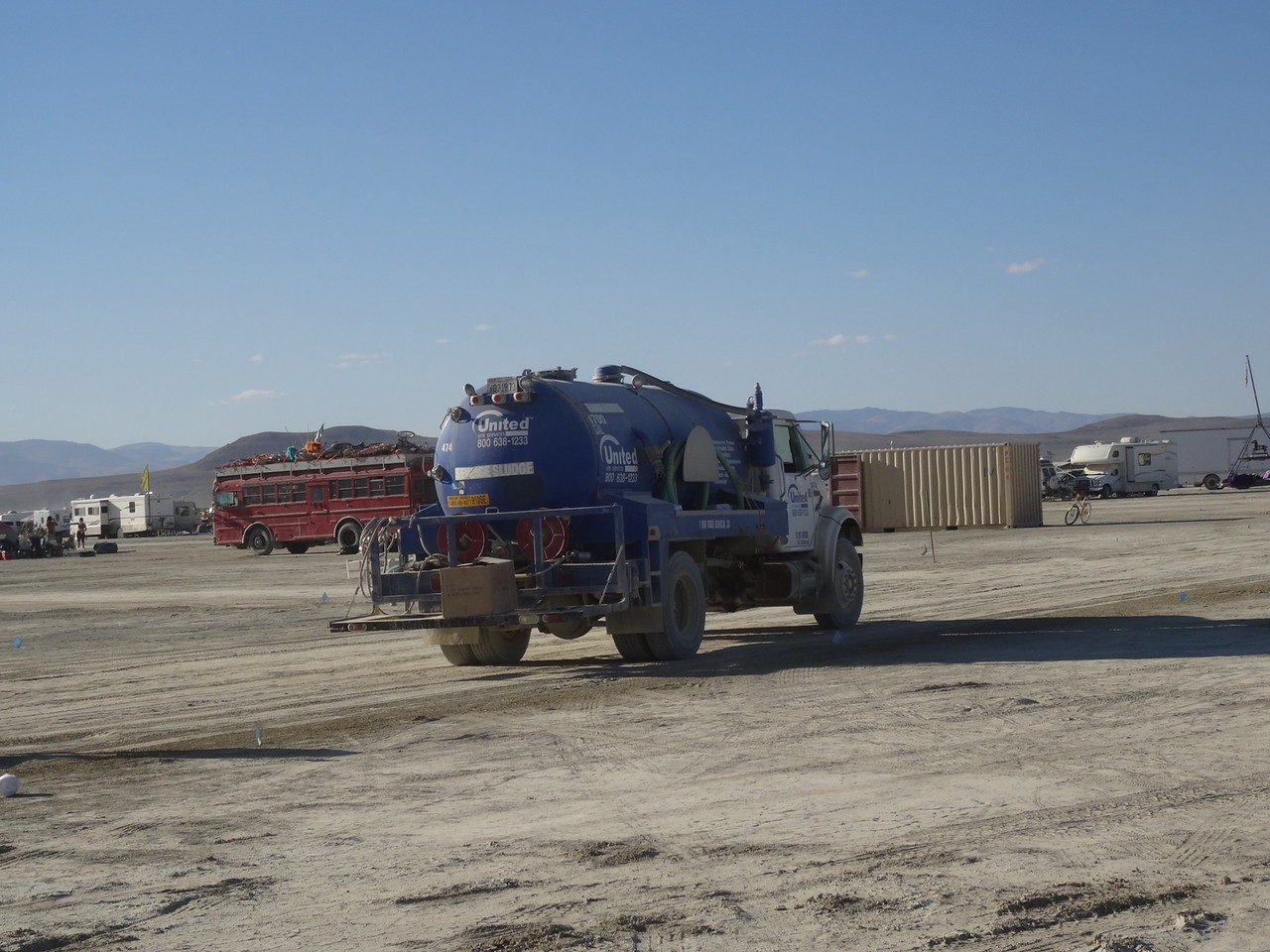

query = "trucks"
[1071,437,1180,499]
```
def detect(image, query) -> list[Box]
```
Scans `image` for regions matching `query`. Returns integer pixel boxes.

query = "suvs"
[1039,458,1060,497]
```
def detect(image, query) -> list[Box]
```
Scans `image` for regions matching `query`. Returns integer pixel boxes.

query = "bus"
[210,448,441,557]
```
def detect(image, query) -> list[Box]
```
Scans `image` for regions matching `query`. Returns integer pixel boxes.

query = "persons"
[77,517,87,551]
[46,516,58,534]
[195,510,209,533]
[209,501,215,513]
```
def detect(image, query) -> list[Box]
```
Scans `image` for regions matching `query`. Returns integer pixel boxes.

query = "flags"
[142,466,148,492]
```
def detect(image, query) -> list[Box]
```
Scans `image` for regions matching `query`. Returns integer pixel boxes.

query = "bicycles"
[1064,491,1092,525]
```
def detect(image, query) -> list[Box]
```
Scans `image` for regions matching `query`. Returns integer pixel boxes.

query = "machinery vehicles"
[327,363,867,665]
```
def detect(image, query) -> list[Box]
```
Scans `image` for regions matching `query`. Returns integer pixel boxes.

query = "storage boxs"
[437,556,519,620]
